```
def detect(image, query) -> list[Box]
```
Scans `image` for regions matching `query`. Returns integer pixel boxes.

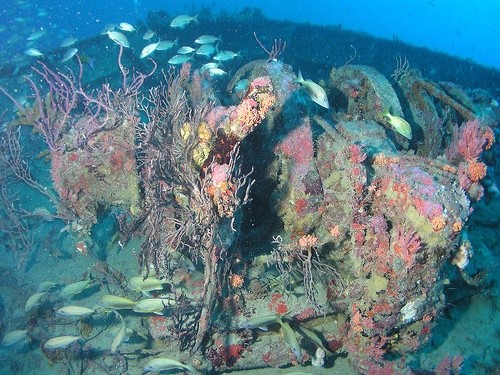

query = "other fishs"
[2,270,335,375]
[380,105,412,140]
[0,0,330,114]
